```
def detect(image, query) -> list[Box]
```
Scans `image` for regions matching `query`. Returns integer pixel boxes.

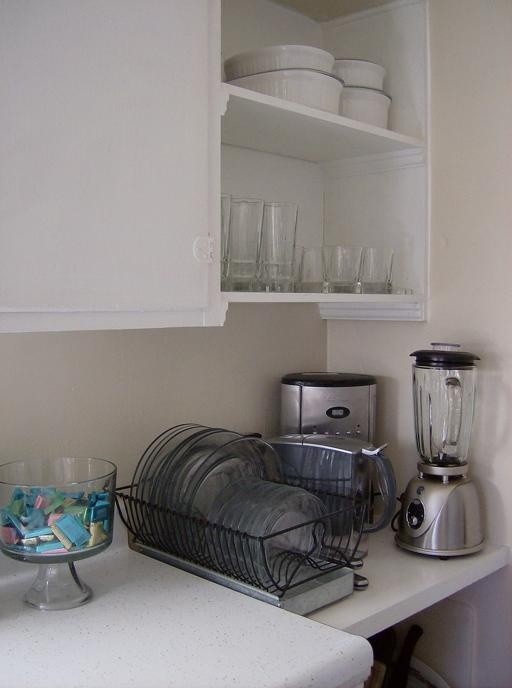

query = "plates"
[131,423,332,591]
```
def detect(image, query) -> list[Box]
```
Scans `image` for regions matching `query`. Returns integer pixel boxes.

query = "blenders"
[393,343,484,557]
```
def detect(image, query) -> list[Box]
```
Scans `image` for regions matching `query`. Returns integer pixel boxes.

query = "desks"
[127,504,512,688]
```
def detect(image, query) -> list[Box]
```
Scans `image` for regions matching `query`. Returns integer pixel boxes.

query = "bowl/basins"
[225,68,344,116]
[333,59,386,89]
[341,86,392,129]
[223,46,335,82]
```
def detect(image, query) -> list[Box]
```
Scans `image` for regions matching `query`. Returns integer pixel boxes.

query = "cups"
[293,244,329,292]
[258,201,300,291]
[227,199,265,292]
[359,245,395,293]
[221,196,231,291]
[325,244,363,291]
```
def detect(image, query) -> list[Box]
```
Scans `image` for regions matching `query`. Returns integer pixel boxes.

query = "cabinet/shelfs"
[222,1,427,322]
[0,1,221,332]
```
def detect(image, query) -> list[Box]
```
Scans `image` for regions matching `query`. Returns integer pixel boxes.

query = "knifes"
[319,555,368,591]
[322,545,367,568]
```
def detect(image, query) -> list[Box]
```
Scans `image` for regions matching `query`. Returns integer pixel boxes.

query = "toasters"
[281,372,377,442]
